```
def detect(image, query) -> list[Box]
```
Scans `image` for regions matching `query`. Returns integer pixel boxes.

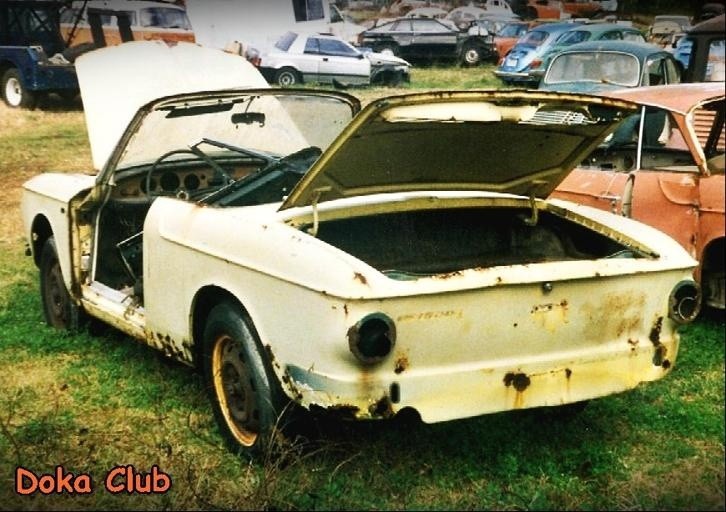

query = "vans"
[0,0,198,113]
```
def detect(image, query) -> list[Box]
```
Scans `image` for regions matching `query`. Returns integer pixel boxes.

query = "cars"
[15,29,706,466]
[544,81,726,325]
